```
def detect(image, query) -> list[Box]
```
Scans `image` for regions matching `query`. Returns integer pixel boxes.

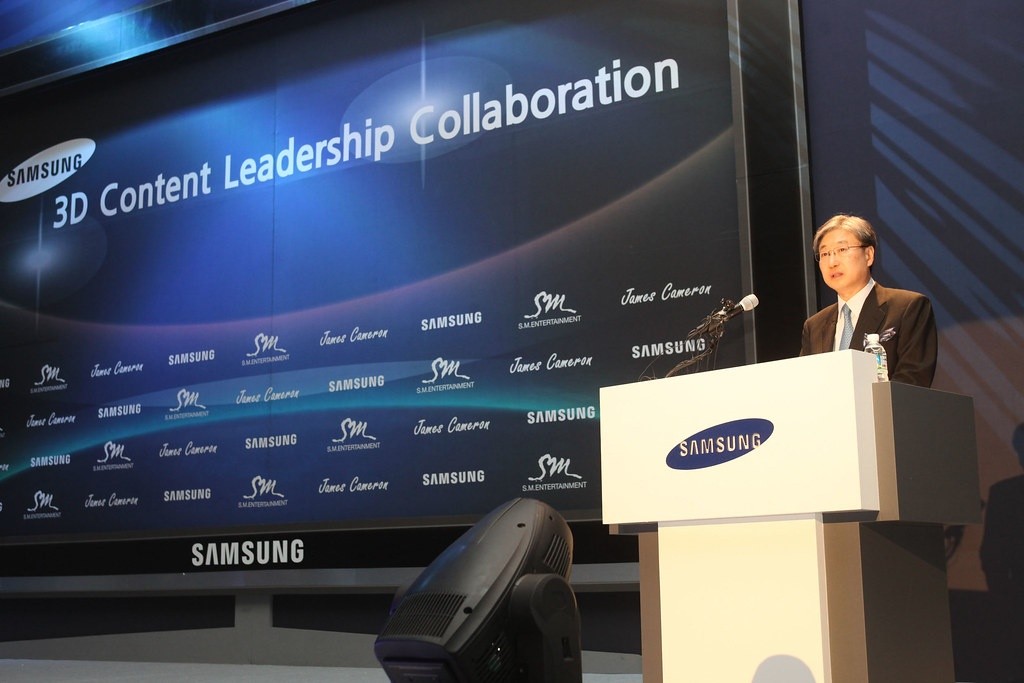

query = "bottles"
[864,333,889,383]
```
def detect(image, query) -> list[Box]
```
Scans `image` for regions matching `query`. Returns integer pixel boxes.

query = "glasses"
[815,244,867,262]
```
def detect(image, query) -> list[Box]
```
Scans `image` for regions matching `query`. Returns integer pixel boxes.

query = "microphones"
[688,293,759,337]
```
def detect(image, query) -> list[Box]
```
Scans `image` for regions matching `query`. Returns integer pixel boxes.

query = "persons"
[800,213,937,390]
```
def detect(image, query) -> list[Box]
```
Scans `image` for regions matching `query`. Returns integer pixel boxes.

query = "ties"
[839,304,855,350]
